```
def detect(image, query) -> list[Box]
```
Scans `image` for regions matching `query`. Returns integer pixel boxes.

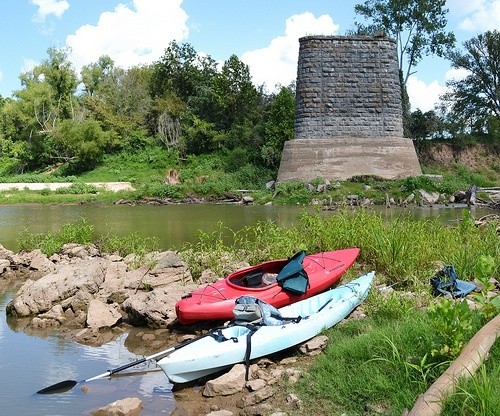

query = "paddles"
[34,326,221,393]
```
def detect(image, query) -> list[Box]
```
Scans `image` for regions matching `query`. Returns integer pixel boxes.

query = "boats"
[156,270,375,384]
[175,247,361,325]
